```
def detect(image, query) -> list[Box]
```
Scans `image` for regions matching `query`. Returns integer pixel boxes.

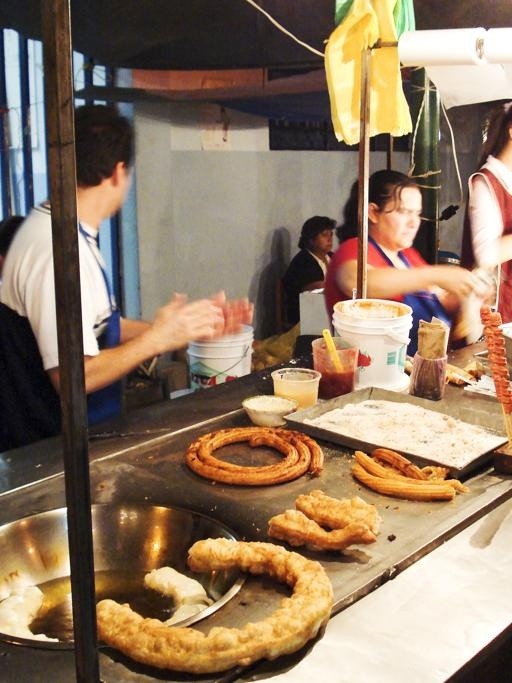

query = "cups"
[271,367,321,413]
[312,336,358,399]
[408,351,448,401]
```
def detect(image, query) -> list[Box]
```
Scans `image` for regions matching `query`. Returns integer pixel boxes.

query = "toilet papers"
[397,26,512,111]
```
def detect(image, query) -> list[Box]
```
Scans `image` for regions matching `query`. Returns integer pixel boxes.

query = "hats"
[298,216,335,247]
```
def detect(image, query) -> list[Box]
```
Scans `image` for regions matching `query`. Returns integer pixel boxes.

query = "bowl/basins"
[242,395,299,427]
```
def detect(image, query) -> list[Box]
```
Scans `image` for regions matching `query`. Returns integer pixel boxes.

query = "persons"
[0,104,256,454]
[457,103,512,326]
[0,214,27,274]
[324,169,495,359]
[278,213,335,332]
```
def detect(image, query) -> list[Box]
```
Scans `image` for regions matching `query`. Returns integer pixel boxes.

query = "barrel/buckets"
[331,297,413,390]
[186,324,255,392]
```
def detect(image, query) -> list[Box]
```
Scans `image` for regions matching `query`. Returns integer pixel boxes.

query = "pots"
[0,502,245,652]
